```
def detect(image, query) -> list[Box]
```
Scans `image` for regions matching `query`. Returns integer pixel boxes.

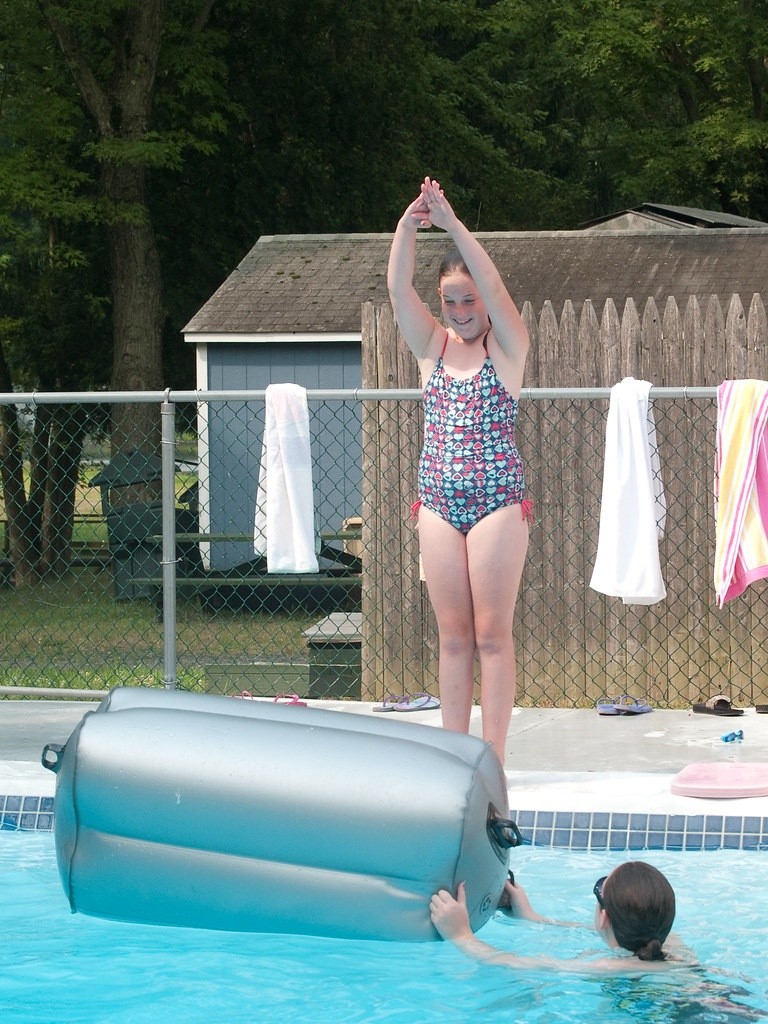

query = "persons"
[429,862,702,975]
[389,175,532,771]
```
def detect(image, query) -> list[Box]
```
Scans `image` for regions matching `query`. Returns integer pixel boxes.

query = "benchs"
[302,611,362,698]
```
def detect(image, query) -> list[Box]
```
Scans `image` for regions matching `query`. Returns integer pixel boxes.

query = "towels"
[713,378,768,611]
[588,376,668,608]
[253,383,320,575]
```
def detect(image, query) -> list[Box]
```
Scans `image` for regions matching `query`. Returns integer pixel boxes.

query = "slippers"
[230,691,307,707]
[597,695,654,716]
[692,694,745,717]
[373,692,440,713]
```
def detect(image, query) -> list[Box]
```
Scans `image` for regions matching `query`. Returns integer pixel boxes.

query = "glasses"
[593,875,609,910]
